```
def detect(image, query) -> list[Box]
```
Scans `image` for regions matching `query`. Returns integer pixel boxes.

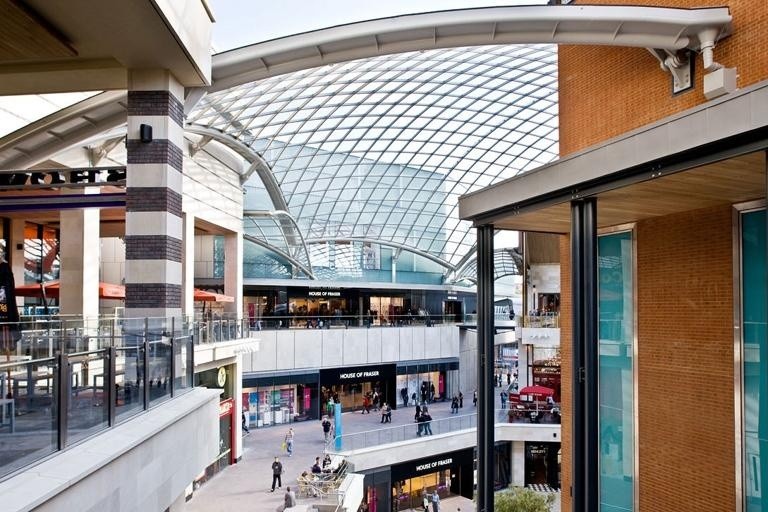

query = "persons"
[294,305,435,329]
[269,455,283,493]
[497,373,501,387]
[241,411,249,434]
[297,454,348,498]
[423,494,429,512]
[283,427,293,458]
[430,490,440,512]
[283,487,296,512]
[420,487,427,508]
[317,380,476,441]
[545,394,554,409]
[499,389,508,409]
[506,372,510,385]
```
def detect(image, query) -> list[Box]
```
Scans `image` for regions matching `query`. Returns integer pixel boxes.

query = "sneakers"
[378,409,380,411]
[456,411,458,413]
[416,432,432,437]
[271,489,274,491]
[380,420,391,423]
[279,487,282,489]
[452,412,454,414]
[374,410,376,411]
[361,412,363,414]
[289,455,291,456]
[368,412,369,413]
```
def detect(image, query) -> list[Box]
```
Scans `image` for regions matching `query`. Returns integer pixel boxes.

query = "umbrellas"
[0,238,22,398]
[519,384,554,416]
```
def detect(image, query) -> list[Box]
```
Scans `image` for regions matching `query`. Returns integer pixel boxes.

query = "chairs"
[0,372,15,433]
[296,477,310,498]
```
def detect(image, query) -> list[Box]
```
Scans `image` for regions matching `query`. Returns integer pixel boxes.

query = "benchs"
[93,369,125,405]
